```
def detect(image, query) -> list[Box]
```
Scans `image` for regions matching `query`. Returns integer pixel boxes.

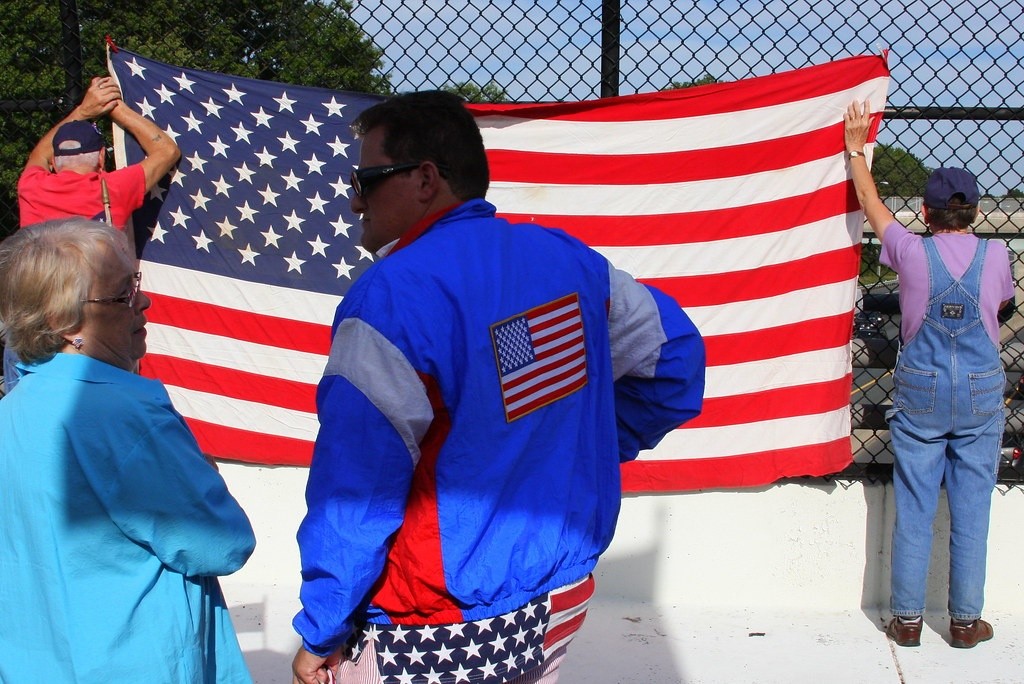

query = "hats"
[923,167,979,209]
[52,121,106,156]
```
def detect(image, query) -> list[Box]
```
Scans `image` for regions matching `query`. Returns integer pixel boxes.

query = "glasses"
[82,271,142,308]
[349,161,448,198]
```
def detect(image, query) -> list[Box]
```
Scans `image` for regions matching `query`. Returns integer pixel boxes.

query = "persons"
[1,217,256,683]
[291,91,708,684]
[843,100,1017,649]
[3,76,182,393]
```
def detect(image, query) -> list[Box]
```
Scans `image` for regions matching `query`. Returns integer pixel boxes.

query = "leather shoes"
[949,619,994,648]
[888,612,922,647]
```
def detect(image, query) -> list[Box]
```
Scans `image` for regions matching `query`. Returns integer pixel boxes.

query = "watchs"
[849,151,865,163]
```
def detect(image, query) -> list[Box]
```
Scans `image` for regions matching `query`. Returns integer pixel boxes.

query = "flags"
[106,30,891,498]
